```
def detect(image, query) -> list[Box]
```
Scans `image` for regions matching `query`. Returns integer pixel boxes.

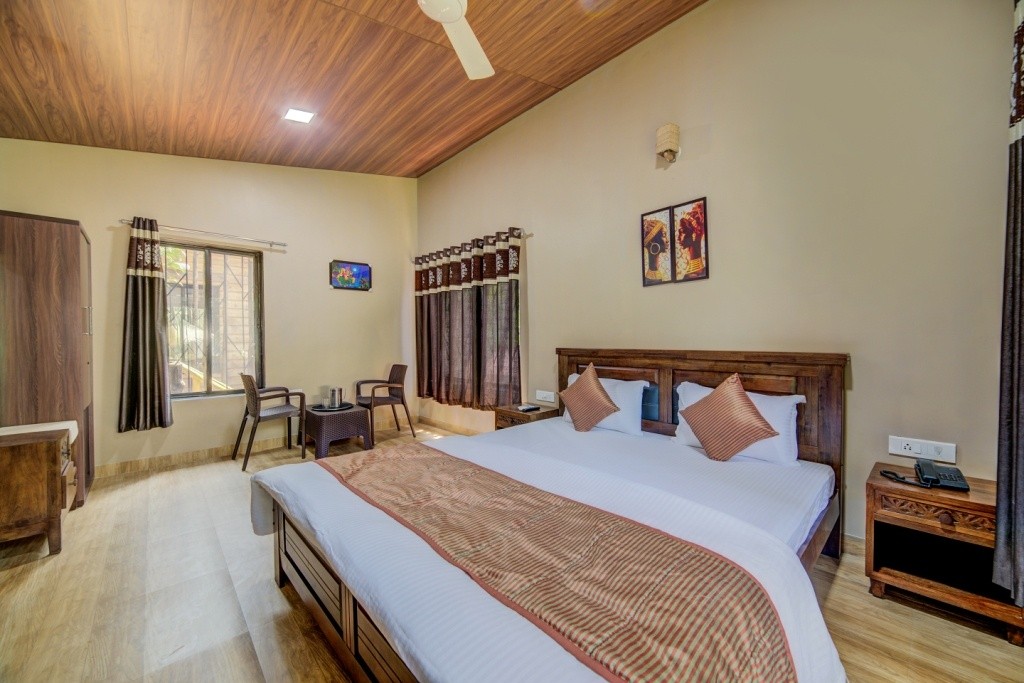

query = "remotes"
[522,406,540,411]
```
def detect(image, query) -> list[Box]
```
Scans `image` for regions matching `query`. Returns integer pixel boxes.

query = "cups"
[322,399,330,410]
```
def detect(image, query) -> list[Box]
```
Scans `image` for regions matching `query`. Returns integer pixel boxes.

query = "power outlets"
[888,435,956,464]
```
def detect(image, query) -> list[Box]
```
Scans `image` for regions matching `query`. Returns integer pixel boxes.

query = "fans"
[418,0,495,80]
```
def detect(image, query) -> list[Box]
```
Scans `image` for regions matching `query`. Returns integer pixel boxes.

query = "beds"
[249,348,849,683]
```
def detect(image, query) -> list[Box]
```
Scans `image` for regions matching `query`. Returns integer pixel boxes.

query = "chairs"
[232,372,306,471]
[356,364,416,447]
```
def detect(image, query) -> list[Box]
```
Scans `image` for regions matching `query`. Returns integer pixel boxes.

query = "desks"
[297,404,373,460]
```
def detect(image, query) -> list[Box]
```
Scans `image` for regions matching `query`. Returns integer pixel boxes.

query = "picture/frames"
[328,259,373,292]
[641,196,709,287]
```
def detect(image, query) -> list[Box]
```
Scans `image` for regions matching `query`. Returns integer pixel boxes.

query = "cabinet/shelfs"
[0,210,94,555]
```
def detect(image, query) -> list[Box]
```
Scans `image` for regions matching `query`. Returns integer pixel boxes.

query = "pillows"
[674,382,807,466]
[557,362,620,432]
[563,372,649,435]
[679,372,780,462]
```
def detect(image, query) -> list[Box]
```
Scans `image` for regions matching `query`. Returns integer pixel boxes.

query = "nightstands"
[865,462,1024,647]
[494,401,559,431]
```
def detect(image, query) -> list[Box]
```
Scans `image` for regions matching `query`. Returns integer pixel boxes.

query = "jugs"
[329,385,342,408]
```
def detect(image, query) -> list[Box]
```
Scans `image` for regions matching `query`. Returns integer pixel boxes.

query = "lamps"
[654,123,681,163]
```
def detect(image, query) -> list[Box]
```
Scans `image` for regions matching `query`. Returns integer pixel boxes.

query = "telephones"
[914,458,970,493]
